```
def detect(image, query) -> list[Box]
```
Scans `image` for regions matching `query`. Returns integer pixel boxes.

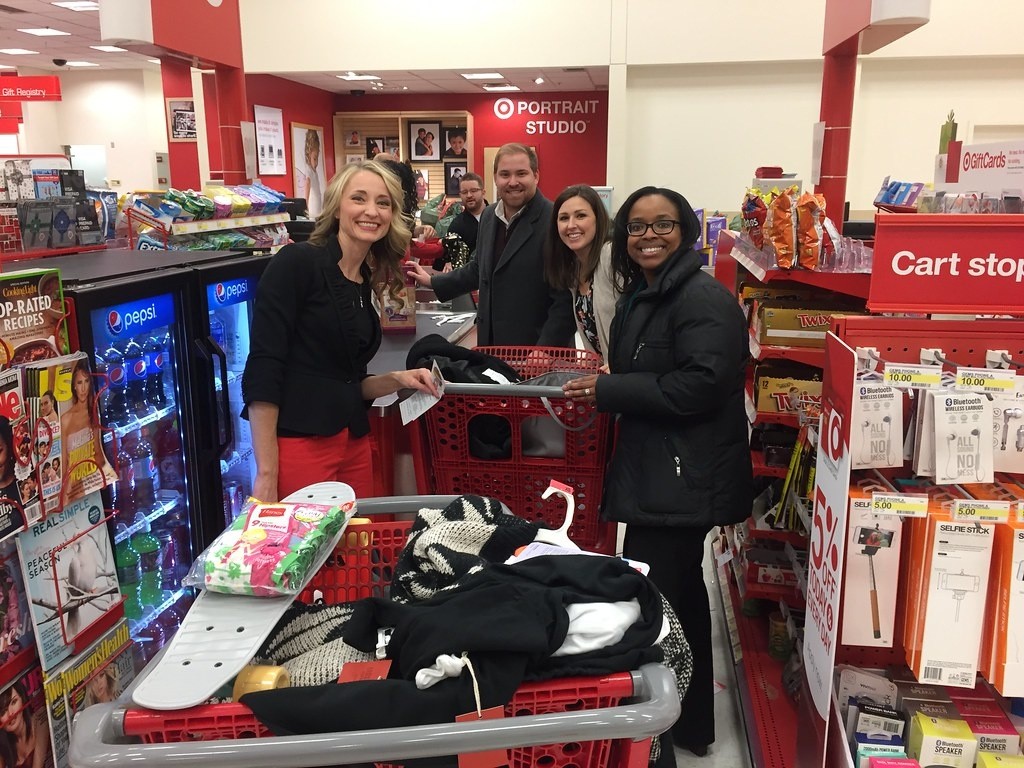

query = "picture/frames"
[407,120,443,162]
[345,130,361,147]
[289,121,328,219]
[385,137,399,158]
[165,97,197,143]
[443,127,467,158]
[444,161,467,195]
[346,154,364,164]
[366,137,384,161]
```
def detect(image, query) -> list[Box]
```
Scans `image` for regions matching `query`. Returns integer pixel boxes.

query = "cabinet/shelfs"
[829,314,1024,768]
[126,206,292,255]
[708,230,874,663]
[0,154,109,261]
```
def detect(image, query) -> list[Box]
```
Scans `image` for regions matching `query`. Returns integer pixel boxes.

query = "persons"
[350,131,359,144]
[39,441,46,457]
[303,129,321,222]
[27,476,39,498]
[374,153,493,274]
[415,132,435,156]
[40,390,60,427]
[61,359,106,505]
[385,296,407,321]
[546,186,634,374]
[405,142,575,372]
[561,186,753,768]
[41,461,56,485]
[450,168,464,191]
[52,457,60,479]
[415,128,432,151]
[445,129,468,158]
[0,415,25,541]
[240,160,439,605]
[20,481,31,503]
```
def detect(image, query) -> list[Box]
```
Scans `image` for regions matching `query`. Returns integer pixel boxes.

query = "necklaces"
[339,263,364,308]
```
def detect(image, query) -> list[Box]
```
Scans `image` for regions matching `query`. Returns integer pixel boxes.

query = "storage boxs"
[733,527,755,556]
[755,167,784,179]
[751,364,823,414]
[379,264,418,334]
[830,374,1024,768]
[3,159,36,201]
[741,549,809,585]
[751,297,862,347]
[0,163,9,200]
[737,279,810,321]
[34,168,62,199]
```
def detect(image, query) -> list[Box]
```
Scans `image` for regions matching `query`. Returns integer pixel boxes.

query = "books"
[0,267,136,768]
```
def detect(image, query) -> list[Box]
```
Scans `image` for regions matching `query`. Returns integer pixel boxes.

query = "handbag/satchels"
[514,370,598,457]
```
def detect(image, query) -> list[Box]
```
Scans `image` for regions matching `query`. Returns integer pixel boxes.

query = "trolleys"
[407,346,617,554]
[69,493,681,768]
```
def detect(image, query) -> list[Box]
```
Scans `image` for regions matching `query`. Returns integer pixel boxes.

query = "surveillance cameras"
[52,58,68,66]
[350,90,365,97]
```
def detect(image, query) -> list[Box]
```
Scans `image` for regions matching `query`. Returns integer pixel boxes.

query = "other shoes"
[671,732,707,757]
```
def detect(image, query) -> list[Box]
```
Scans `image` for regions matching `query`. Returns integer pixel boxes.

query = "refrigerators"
[0,249,272,669]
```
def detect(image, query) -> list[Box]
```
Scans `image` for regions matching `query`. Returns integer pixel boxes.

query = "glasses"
[625,219,681,236]
[461,188,482,198]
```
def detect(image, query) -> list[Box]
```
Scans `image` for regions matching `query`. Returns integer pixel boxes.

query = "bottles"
[94,309,230,675]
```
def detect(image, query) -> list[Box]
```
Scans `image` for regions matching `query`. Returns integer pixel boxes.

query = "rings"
[585,388,591,396]
